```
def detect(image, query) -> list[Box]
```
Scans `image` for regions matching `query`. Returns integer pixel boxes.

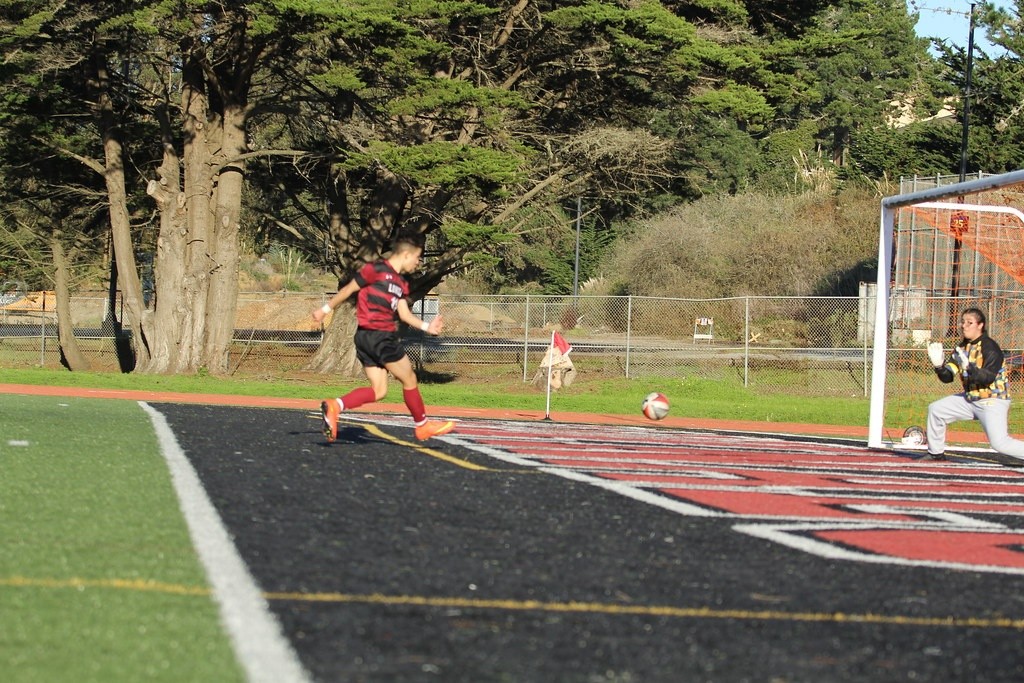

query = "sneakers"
[321,397,339,444]
[414,419,455,441]
[915,455,947,462]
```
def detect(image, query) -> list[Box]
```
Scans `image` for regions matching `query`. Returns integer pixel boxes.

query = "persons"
[311,237,456,442]
[912,307,1024,462]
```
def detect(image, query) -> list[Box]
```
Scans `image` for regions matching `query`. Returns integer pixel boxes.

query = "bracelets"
[420,321,430,332]
[321,304,332,314]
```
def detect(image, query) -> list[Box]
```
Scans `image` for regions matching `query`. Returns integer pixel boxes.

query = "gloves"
[928,342,944,369]
[951,347,969,372]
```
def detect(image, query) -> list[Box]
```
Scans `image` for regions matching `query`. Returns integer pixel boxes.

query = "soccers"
[641,392,670,420]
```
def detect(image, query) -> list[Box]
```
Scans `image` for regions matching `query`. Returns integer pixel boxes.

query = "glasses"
[961,320,979,326]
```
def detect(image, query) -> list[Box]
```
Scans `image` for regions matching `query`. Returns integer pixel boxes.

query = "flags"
[553,331,572,357]
